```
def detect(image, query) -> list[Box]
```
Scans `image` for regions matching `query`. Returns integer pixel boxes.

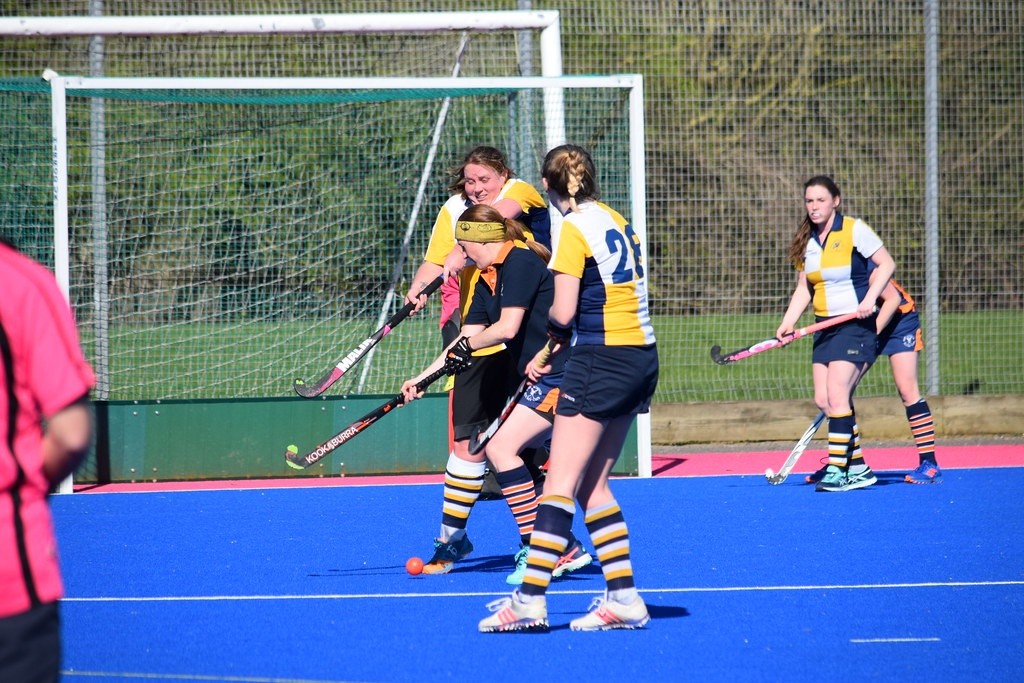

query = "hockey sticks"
[293,257,476,398]
[764,411,826,485]
[467,338,559,455]
[710,304,878,366]
[284,366,445,471]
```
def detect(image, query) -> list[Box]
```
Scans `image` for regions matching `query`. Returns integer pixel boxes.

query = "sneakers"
[422,532,473,575]
[551,540,593,580]
[506,545,529,585]
[904,460,943,485]
[570,587,650,632]
[478,587,549,633]
[805,456,829,482]
[845,465,878,490]
[816,465,849,491]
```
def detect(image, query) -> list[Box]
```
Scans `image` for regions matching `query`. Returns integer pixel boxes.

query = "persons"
[401,145,658,633]
[776,176,942,493]
[0,236,96,683]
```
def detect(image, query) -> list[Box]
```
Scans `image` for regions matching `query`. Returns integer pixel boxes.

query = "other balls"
[406,557,424,575]
